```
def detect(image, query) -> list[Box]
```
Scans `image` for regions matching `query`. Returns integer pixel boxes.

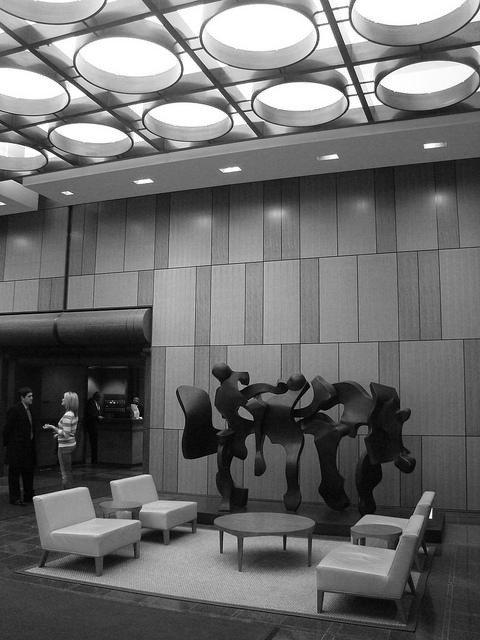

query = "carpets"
[13,523,437,633]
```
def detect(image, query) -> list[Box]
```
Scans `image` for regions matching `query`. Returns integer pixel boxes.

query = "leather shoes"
[12,499,32,505]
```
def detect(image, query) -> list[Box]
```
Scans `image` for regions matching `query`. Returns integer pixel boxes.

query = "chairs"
[32,473,198,577]
[315,489,437,625]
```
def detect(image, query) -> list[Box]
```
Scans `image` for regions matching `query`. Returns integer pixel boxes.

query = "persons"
[133,397,144,419]
[84,391,105,464]
[2,386,37,506]
[42,392,80,490]
